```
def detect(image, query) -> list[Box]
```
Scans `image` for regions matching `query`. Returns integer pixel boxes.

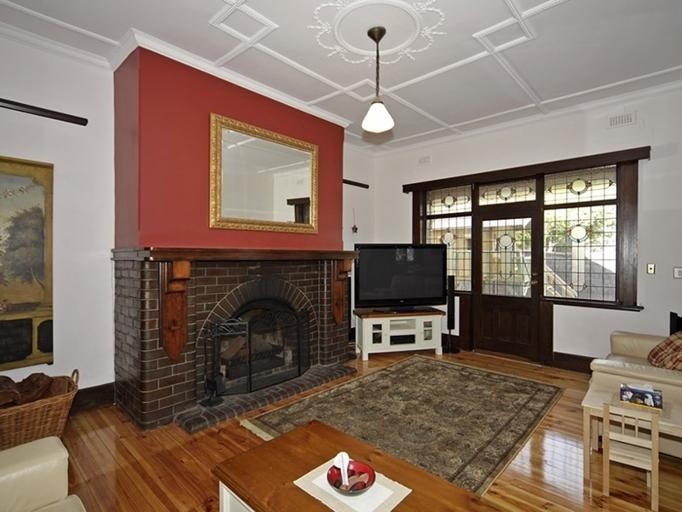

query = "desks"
[210,419,519,512]
[581,381,682,481]
[353,306,446,361]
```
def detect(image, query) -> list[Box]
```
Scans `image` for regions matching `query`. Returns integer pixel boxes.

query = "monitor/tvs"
[353,241,448,314]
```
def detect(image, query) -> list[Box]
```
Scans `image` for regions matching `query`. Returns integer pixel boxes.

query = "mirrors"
[208,111,322,236]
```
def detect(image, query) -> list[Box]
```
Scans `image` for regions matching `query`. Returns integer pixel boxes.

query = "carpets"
[240,354,567,497]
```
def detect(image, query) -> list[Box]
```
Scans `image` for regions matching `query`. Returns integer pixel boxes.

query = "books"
[618,383,663,415]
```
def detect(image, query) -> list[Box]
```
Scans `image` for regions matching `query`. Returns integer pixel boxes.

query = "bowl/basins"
[327,461,375,496]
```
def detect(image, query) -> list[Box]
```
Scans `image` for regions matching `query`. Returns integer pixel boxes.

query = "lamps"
[361,25,395,135]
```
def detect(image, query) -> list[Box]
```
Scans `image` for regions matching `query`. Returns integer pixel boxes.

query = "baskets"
[0,369,81,452]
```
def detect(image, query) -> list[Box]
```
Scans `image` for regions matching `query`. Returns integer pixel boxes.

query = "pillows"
[648,331,682,371]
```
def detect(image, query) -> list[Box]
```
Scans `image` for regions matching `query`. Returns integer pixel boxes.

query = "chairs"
[590,329,682,424]
[0,435,88,512]
[601,402,660,512]
